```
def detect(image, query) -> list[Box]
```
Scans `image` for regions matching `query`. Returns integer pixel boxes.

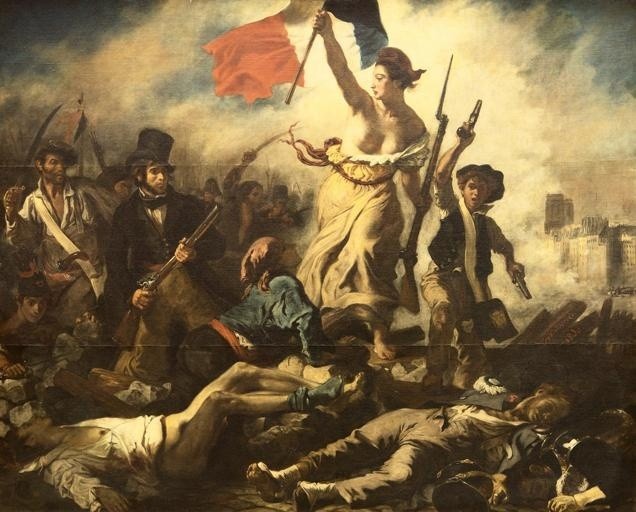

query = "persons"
[1,9,635,512]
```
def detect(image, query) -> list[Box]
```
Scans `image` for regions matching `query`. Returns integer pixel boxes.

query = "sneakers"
[247,462,285,504]
[293,480,309,511]
[288,376,345,413]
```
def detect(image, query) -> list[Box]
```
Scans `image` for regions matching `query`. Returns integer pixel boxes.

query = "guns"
[513,270,532,300]
[457,97,484,138]
[112,203,223,351]
[399,52,454,315]
[57,252,88,278]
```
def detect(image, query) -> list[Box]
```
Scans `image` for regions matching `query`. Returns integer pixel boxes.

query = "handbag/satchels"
[473,299,518,343]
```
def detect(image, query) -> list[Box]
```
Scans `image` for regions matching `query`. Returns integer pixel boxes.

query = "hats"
[20,271,50,296]
[34,141,76,167]
[457,165,505,203]
[433,460,494,511]
[126,127,177,173]
